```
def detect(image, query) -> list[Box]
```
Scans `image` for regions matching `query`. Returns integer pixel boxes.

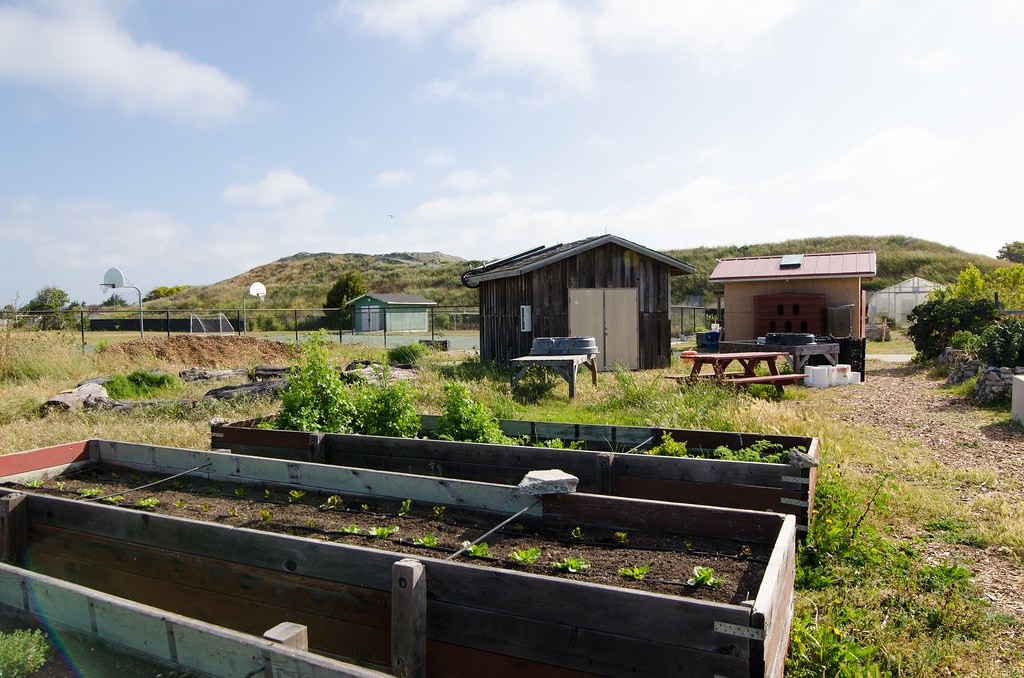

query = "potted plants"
[0,326,821,678]
[936,313,1024,404]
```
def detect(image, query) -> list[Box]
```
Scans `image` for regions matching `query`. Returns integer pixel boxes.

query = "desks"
[679,352,790,367]
[509,353,598,398]
[725,344,840,385]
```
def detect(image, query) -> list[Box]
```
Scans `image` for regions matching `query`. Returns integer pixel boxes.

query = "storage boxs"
[803,363,862,391]
[696,331,719,354]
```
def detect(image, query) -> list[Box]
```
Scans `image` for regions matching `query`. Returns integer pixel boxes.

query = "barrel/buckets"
[804,364,861,388]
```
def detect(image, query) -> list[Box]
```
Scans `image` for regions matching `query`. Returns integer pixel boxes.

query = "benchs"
[663,371,809,388]
